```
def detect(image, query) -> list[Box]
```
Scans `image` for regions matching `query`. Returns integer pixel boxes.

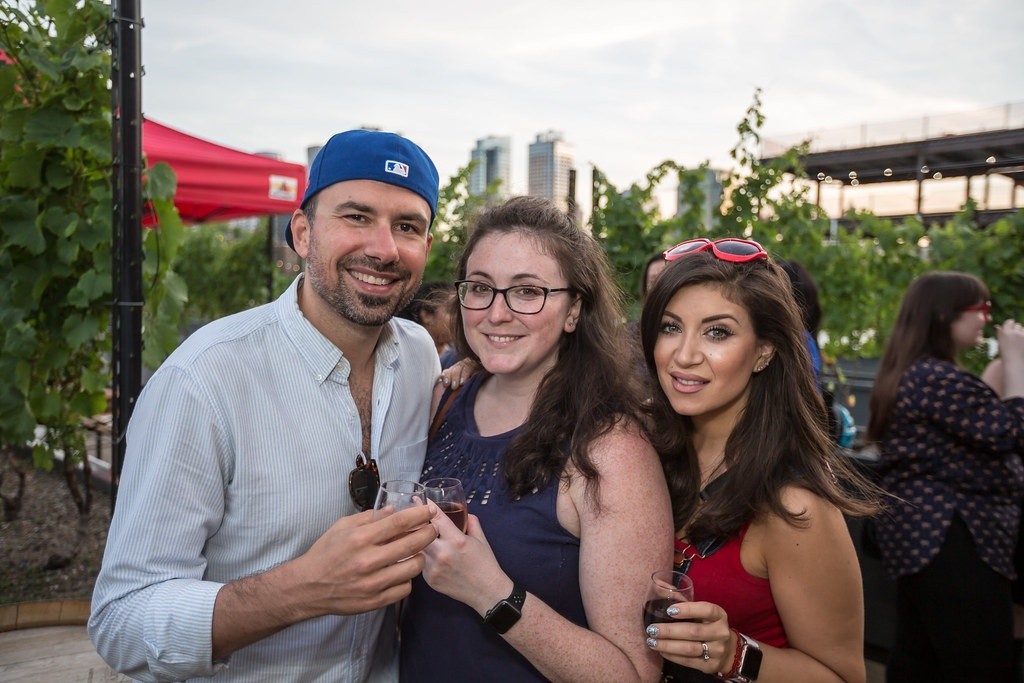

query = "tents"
[138,112,305,230]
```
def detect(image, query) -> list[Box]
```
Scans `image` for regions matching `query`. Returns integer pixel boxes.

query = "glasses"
[454,281,574,314]
[956,300,991,320]
[663,238,770,271]
[348,455,380,512]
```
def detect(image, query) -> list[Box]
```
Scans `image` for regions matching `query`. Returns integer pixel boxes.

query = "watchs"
[481,581,527,635]
[727,631,764,682]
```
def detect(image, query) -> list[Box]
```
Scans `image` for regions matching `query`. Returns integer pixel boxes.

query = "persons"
[869,270,1024,683]
[400,197,903,683]
[85,128,442,683]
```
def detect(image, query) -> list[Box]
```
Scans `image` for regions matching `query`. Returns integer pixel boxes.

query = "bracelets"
[718,625,743,681]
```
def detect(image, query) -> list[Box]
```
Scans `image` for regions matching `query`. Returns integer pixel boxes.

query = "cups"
[421,478,468,535]
[642,570,694,637]
[373,479,427,565]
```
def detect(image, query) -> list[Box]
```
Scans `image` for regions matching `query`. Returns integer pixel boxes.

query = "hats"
[285,130,439,254]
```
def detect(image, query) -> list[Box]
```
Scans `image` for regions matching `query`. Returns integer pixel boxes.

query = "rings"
[702,641,710,661]
